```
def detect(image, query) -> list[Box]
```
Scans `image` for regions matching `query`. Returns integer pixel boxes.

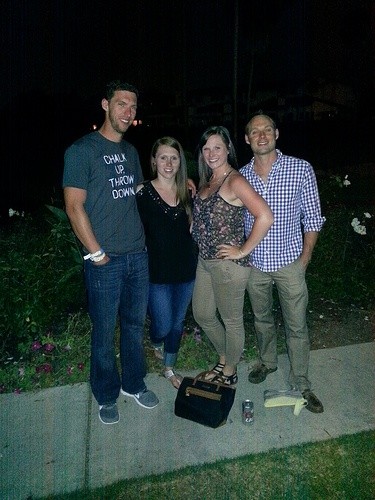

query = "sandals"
[213,370,237,386]
[153,342,164,360]
[206,362,225,381]
[165,368,183,390]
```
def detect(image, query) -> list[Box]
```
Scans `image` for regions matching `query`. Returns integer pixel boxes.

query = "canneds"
[242,399,255,425]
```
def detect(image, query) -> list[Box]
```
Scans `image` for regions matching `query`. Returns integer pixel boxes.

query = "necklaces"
[207,165,232,189]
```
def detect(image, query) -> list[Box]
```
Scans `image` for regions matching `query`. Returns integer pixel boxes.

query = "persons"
[238,111,326,413]
[63,82,197,425]
[136,138,196,389]
[185,124,275,389]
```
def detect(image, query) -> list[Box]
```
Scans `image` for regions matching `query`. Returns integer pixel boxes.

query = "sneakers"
[99,401,120,424]
[121,386,159,409]
[249,364,278,383]
[302,389,323,414]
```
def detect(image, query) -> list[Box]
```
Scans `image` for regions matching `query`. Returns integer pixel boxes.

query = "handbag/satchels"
[174,370,236,429]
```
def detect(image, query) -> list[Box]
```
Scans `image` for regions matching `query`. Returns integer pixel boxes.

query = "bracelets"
[83,249,106,262]
